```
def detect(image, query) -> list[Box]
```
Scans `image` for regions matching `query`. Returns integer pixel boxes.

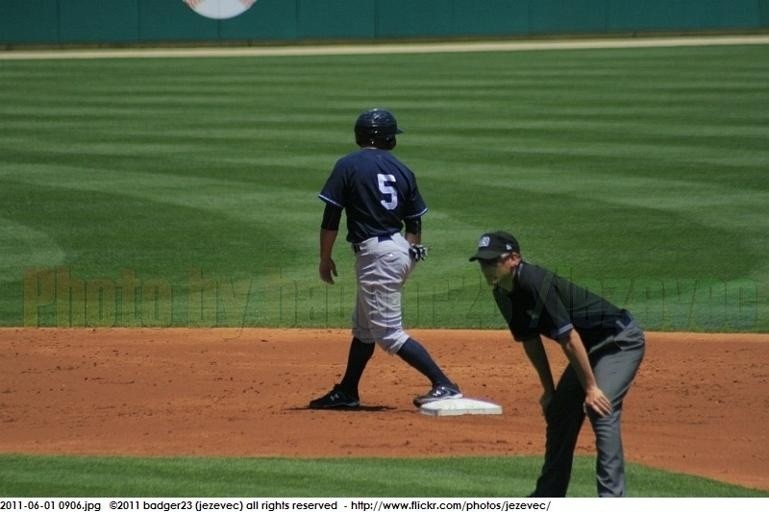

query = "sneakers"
[309,383,361,409]
[413,381,464,407]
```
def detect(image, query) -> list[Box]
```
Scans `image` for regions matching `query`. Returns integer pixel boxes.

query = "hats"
[469,230,520,262]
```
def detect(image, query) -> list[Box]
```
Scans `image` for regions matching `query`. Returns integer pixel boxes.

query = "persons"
[310,108,464,410]
[469,231,646,497]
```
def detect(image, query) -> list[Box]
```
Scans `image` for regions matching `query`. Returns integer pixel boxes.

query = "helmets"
[354,109,404,150]
